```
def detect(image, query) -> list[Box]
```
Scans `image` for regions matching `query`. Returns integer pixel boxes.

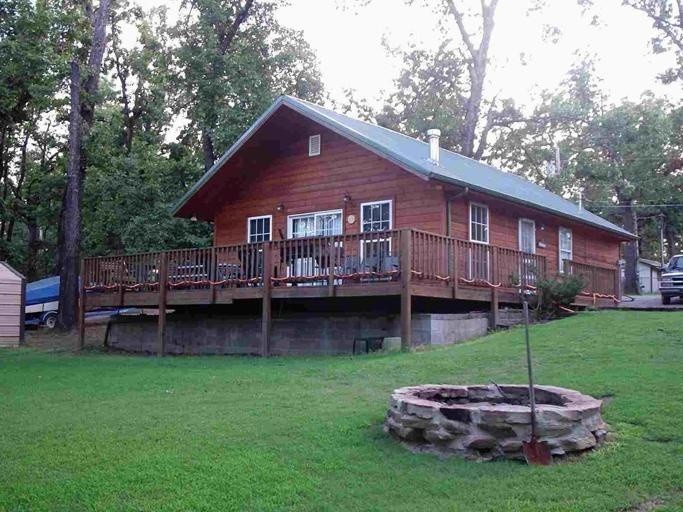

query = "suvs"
[658,254,683,304]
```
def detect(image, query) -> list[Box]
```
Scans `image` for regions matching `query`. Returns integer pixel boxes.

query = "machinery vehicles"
[22,276,59,331]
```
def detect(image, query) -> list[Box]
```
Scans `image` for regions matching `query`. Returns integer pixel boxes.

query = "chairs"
[340,255,400,284]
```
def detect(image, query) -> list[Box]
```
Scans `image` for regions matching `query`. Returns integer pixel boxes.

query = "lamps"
[342,190,353,206]
[276,201,284,211]
